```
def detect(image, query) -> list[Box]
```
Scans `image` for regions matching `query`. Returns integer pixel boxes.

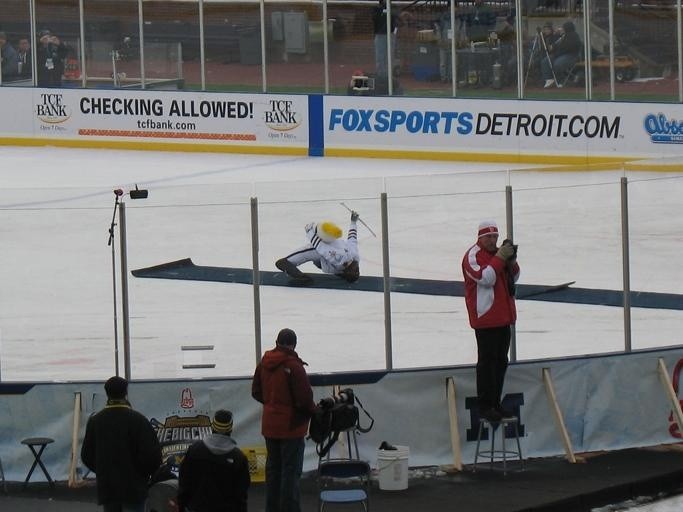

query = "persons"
[0,20,71,88]
[81,377,161,512]
[275,210,360,283]
[369,2,582,90]
[177,409,250,511]
[252,327,323,511]
[461,220,521,422]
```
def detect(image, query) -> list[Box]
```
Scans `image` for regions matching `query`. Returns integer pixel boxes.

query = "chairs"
[315,457,371,512]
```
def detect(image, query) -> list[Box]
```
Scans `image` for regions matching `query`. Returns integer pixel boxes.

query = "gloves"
[495,242,515,262]
[350,210,359,223]
[506,254,518,266]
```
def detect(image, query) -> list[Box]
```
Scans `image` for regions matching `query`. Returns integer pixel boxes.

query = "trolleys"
[574,54,639,82]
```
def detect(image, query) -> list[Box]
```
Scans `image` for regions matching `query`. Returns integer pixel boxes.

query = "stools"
[471,416,525,477]
[324,425,366,488]
[20,437,56,492]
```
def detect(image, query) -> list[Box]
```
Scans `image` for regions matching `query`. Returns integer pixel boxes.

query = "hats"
[209,408,235,435]
[476,220,499,238]
[276,328,297,346]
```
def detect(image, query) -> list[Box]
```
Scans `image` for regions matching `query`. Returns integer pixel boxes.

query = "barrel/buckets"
[375,34,399,79]
[376,445,410,491]
[411,42,439,80]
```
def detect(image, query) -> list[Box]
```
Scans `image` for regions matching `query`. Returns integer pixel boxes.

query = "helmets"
[341,260,359,284]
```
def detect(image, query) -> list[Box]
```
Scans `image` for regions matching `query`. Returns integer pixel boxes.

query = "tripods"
[524,28,559,89]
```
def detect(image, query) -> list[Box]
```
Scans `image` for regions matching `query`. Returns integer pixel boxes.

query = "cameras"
[503,239,518,254]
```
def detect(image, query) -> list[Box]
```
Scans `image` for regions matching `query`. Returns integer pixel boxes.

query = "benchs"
[571,58,641,70]
[2,2,377,58]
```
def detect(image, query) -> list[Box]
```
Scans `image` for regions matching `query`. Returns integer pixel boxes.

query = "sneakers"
[473,399,509,422]
[287,276,314,287]
[544,79,555,88]
